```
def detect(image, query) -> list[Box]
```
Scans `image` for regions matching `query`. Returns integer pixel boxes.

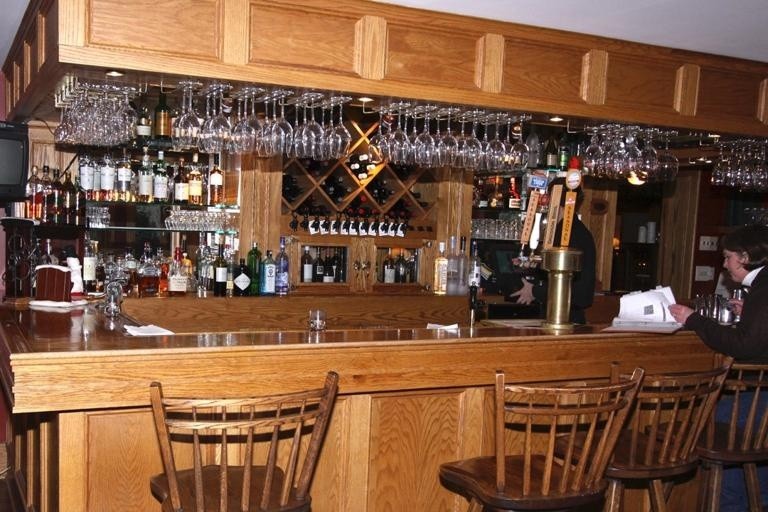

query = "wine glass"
[53,80,144,153]
[739,206,766,228]
[373,98,532,174]
[583,124,767,195]
[172,80,353,162]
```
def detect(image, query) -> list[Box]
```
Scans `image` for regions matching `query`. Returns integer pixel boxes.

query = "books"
[599,285,684,335]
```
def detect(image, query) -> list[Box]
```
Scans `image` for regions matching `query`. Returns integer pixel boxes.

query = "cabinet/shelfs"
[77,134,239,236]
[473,169,535,245]
[282,103,437,222]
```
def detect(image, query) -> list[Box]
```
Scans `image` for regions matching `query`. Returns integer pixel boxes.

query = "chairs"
[439,366,648,511]
[149,370,339,511]
[550,351,734,512]
[645,351,767,512]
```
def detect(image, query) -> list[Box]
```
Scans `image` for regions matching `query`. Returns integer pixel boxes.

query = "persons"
[471,236,508,297]
[508,177,599,325]
[668,224,767,511]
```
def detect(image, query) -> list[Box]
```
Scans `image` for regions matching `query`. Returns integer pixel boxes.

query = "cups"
[9,200,25,220]
[307,309,330,331]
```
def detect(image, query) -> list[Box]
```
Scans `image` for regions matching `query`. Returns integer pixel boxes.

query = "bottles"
[637,224,648,244]
[145,331,476,347]
[469,278,486,330]
[647,220,658,244]
[24,164,42,220]
[533,124,583,174]
[40,163,52,221]
[50,169,62,222]
[690,288,748,329]
[22,174,548,320]
